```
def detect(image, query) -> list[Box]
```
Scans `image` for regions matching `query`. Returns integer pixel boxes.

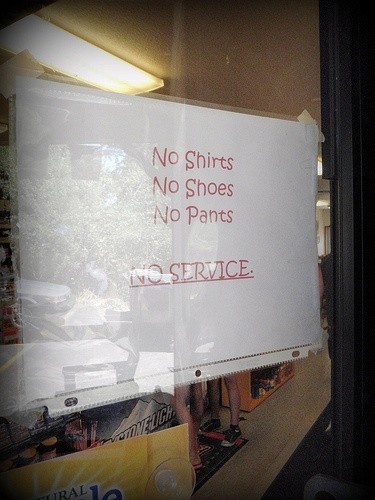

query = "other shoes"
[221,429,242,447]
[200,419,221,433]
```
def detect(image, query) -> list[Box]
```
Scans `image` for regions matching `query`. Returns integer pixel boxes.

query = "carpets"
[188,422,248,496]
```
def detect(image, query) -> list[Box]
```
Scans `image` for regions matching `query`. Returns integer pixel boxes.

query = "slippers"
[193,464,203,470]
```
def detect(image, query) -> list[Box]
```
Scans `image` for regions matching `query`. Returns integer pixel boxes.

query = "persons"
[173,382,205,472]
[199,374,240,448]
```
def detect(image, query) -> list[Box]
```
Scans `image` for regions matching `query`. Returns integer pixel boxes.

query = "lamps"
[0,13,166,98]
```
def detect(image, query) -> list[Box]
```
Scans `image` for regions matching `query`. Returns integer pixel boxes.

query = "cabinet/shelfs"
[221,361,295,412]
